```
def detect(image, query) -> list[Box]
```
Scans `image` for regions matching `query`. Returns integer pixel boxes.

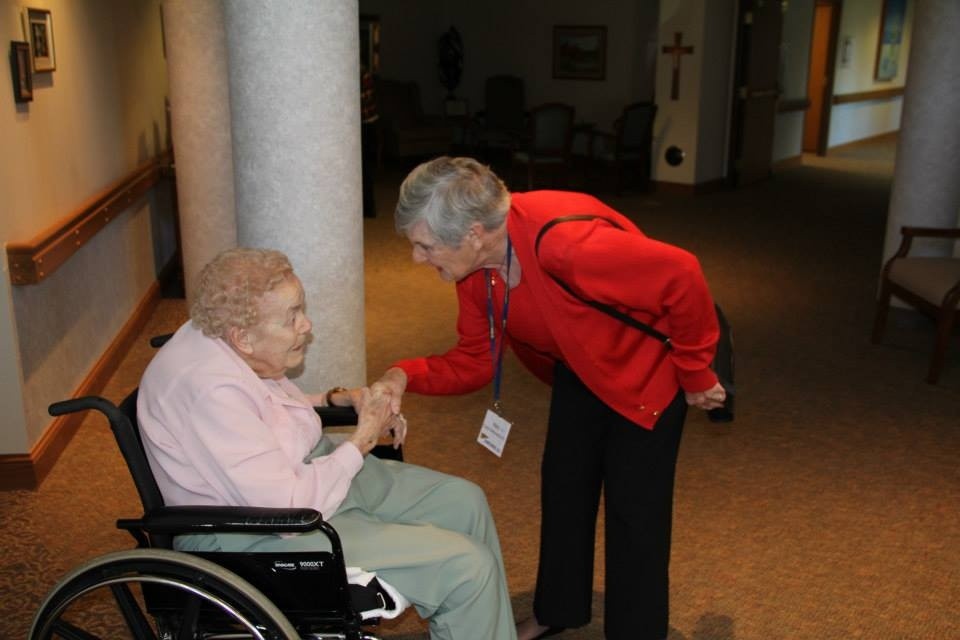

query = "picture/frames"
[552,25,609,80]
[23,7,56,73]
[11,40,33,102]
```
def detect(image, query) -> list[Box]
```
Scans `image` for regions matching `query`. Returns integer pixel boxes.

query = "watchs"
[327,386,348,406]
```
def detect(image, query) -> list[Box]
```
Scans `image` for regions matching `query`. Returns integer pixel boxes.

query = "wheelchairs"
[26,334,431,640]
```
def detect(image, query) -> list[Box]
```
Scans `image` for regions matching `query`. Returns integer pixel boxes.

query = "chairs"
[483,77,657,196]
[872,226,960,383]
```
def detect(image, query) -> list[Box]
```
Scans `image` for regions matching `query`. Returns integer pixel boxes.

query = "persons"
[370,157,729,640]
[136,245,519,640]
